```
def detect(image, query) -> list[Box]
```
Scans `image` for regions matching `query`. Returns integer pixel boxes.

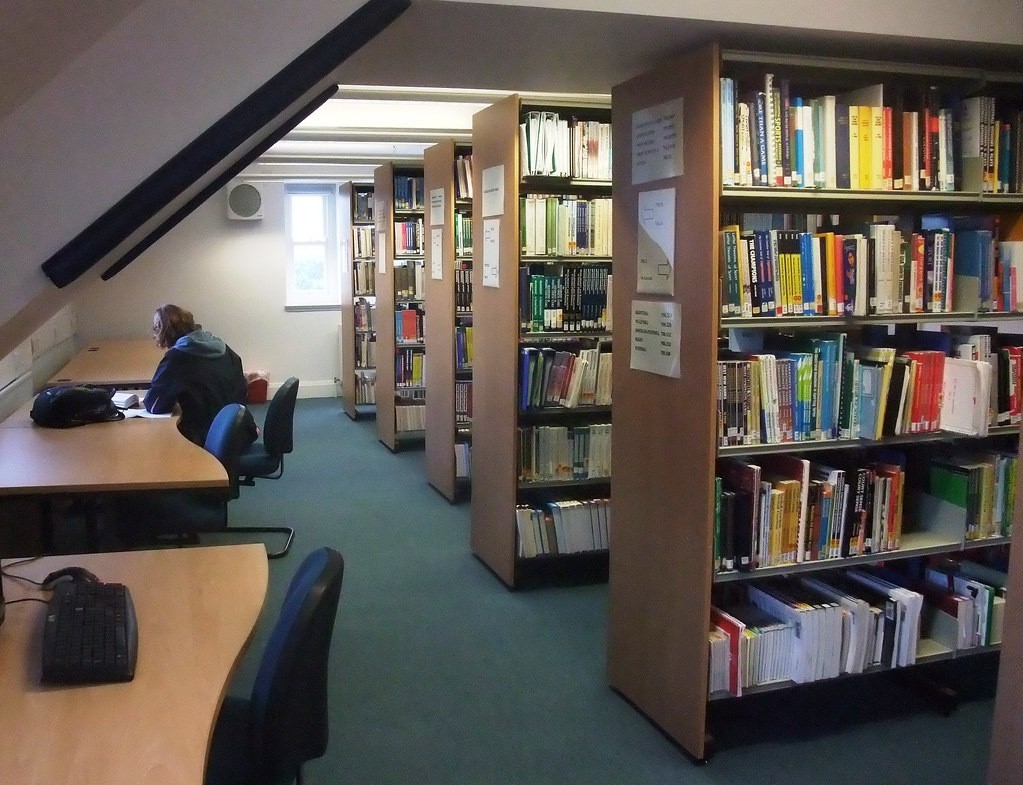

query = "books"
[454,319,474,370]
[112,393,179,419]
[717,329,1023,447]
[455,381,472,421]
[455,261,473,312]
[352,174,426,433]
[520,111,613,181]
[454,154,473,200]
[709,452,1015,697]
[455,440,472,477]
[718,209,1023,319]
[519,338,613,409]
[517,421,612,483]
[455,208,473,259]
[519,193,613,257]
[518,264,613,333]
[720,73,1023,194]
[516,495,611,559]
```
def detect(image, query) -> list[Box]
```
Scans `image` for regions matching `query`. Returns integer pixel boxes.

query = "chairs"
[238,376,300,488]
[115,404,294,559]
[204,547,345,785]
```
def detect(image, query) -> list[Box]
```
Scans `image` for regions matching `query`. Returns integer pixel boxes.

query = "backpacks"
[30,384,125,427]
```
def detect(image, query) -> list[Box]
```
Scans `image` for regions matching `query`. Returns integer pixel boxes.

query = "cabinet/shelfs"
[340,43,1021,761]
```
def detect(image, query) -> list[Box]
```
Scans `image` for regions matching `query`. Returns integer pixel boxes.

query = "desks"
[0,544,268,784]
[42,341,169,384]
[0,389,230,553]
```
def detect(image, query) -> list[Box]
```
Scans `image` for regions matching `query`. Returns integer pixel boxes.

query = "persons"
[143,304,259,533]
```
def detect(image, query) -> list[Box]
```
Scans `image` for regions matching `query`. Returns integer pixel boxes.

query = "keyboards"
[39,580,138,684]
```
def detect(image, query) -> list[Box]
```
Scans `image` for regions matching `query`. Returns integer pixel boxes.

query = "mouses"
[40,566,99,590]
